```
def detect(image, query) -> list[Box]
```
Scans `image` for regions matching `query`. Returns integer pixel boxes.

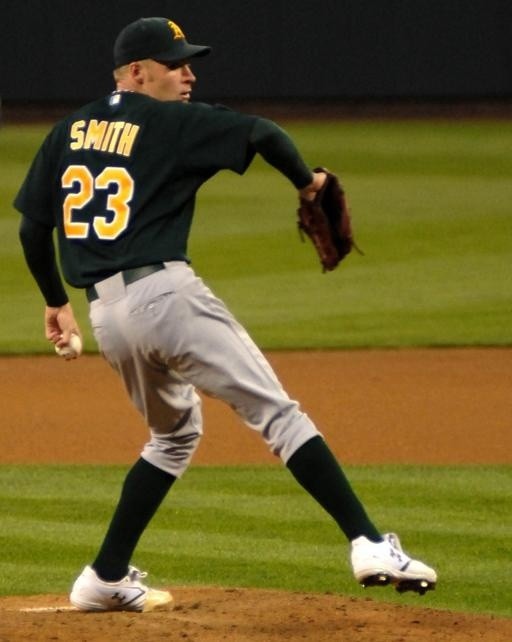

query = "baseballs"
[55,332,82,358]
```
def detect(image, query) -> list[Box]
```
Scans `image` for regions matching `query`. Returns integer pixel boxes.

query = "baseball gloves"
[298,167,352,271]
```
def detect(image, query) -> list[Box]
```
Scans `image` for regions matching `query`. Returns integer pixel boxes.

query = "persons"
[11,16,438,614]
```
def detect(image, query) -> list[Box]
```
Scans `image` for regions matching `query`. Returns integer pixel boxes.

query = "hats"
[112,16,212,75]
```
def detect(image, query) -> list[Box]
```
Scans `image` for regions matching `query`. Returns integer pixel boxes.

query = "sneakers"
[348,533,437,597]
[69,562,177,614]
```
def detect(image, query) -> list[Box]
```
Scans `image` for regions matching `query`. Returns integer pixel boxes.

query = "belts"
[85,263,165,305]
